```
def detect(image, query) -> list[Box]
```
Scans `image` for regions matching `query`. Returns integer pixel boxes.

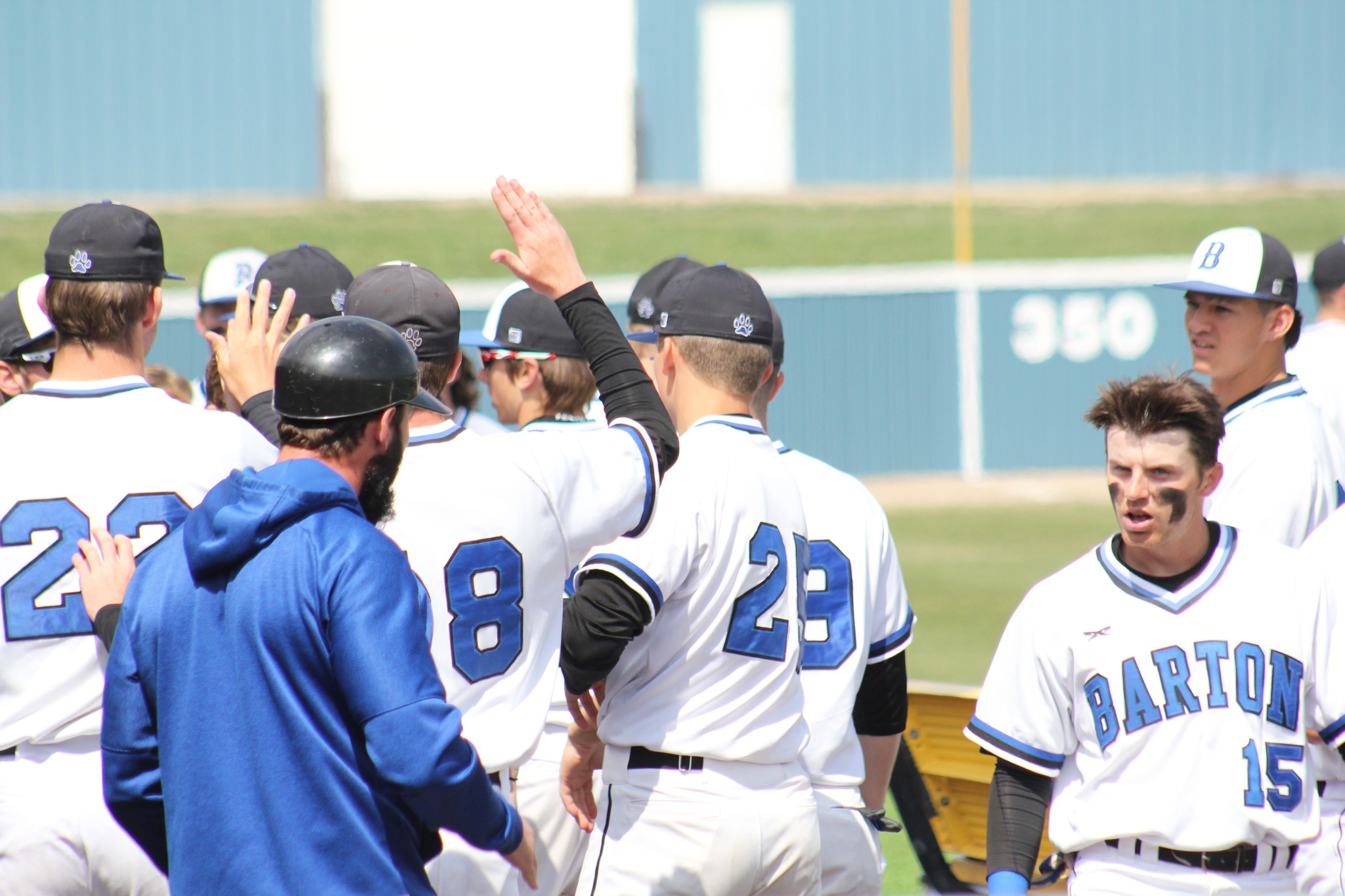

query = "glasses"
[478,348,557,370]
[0,347,57,373]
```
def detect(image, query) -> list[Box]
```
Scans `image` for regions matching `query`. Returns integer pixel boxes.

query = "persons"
[0,175,709,896]
[960,364,1345,896]
[100,315,539,896]
[1153,224,1345,896]
[560,262,917,896]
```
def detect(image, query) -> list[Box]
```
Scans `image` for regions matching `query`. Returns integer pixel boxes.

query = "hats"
[219,244,354,322]
[627,256,706,325]
[1312,238,1345,285]
[769,307,783,365]
[459,279,587,359]
[45,200,187,281]
[627,262,773,345]
[1152,227,1298,309]
[199,246,270,306]
[344,260,460,357]
[0,272,54,358]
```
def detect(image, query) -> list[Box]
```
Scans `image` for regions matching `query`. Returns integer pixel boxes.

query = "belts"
[626,746,704,772]
[1105,838,1299,874]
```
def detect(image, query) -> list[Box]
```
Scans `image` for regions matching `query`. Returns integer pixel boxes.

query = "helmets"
[270,315,453,419]
